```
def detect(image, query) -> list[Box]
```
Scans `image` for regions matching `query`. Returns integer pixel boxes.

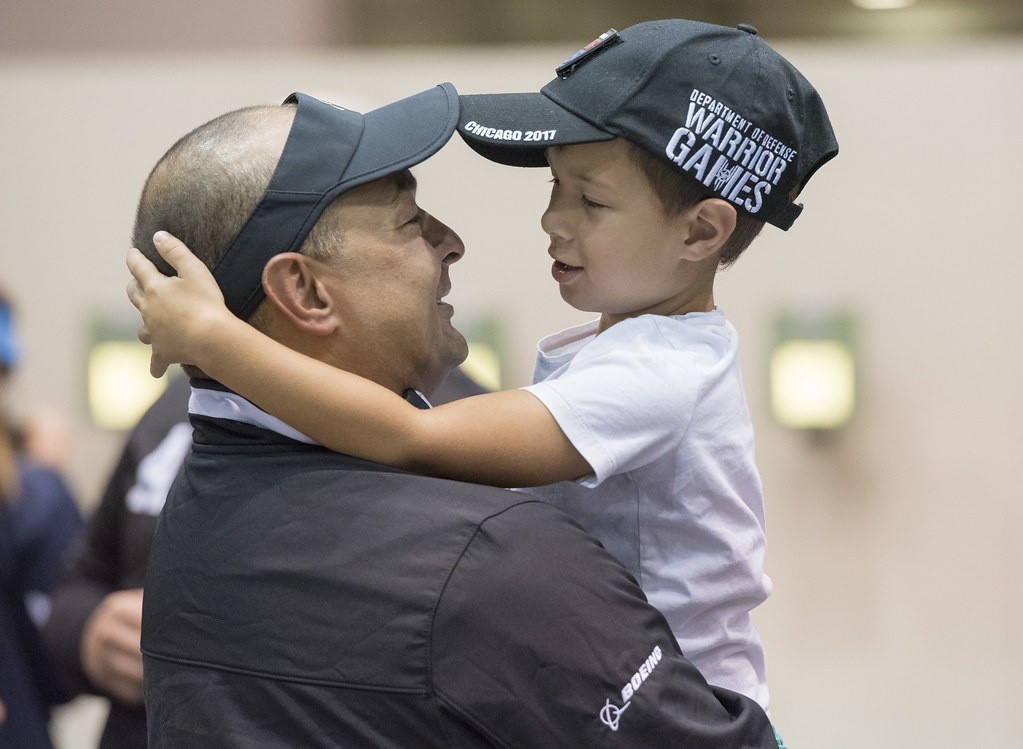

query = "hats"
[454,19,837,232]
[206,84,462,317]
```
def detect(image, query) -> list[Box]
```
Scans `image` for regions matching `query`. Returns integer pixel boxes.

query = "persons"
[0,297,82,749]
[24,19,839,749]
